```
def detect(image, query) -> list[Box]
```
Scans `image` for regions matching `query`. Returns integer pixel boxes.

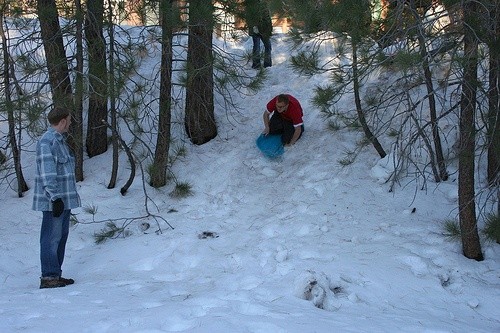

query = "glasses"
[276,104,287,110]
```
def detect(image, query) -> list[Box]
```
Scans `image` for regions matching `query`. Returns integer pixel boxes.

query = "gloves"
[52,198,64,217]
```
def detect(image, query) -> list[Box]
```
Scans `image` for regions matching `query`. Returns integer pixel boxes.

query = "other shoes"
[40,275,74,289]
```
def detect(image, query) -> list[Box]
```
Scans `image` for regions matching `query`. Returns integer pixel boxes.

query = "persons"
[245,0,272,69]
[32,105,82,287]
[263,93,304,147]
